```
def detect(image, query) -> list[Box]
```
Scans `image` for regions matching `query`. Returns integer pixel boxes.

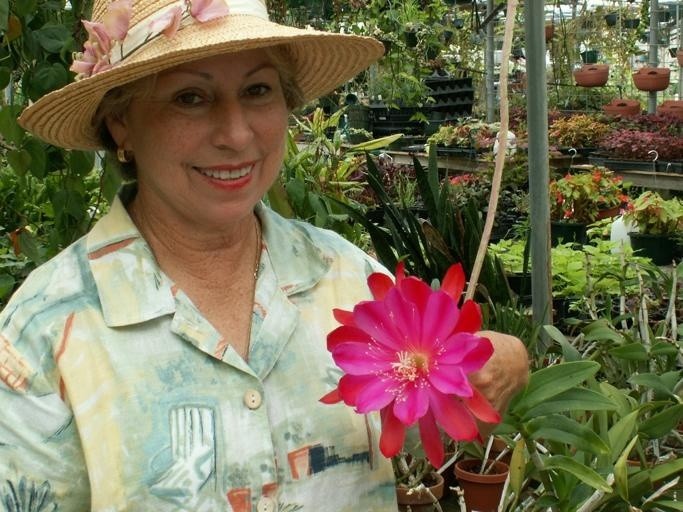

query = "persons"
[0,0,529,511]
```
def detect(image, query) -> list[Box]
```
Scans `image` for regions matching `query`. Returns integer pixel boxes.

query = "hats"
[16,0,385,148]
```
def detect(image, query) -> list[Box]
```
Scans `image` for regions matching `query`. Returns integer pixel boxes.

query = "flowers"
[313,257,505,472]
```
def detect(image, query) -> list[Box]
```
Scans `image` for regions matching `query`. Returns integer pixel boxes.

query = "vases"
[384,452,511,512]
[312,0,683,351]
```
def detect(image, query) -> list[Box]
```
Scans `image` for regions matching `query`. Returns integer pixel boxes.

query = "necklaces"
[252,213,261,283]
